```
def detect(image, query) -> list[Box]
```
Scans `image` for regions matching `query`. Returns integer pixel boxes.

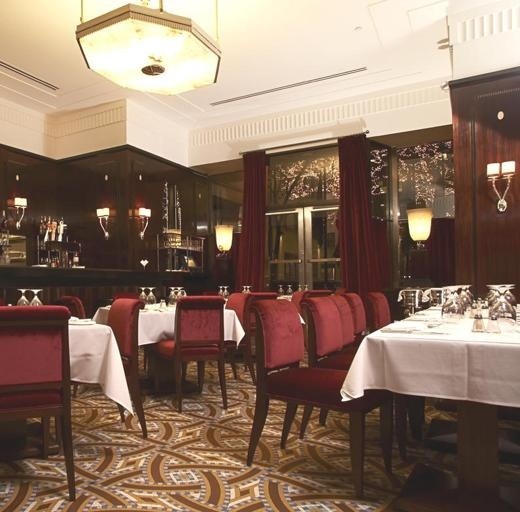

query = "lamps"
[407,207,433,250]
[15,198,28,229]
[215,224,233,255]
[139,207,152,239]
[96,208,110,239]
[76,0,222,95]
[486,160,515,212]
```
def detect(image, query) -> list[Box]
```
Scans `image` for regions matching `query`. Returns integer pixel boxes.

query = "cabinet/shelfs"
[156,234,206,274]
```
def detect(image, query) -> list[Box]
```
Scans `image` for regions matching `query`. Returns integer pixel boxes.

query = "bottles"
[471,311,502,334]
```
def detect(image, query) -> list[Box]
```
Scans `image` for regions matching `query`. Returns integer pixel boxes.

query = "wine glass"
[397,281,519,318]
[137,283,312,308]
[15,288,43,307]
